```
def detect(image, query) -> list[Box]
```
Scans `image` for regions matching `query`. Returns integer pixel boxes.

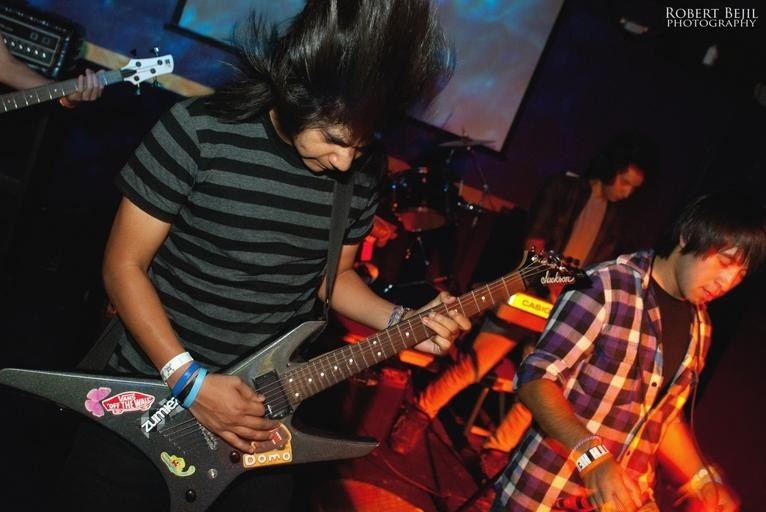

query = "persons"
[523,153,661,269]
[0,37,112,113]
[466,186,766,512]
[49,1,476,512]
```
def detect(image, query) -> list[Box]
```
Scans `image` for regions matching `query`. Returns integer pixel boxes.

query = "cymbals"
[438,139,495,146]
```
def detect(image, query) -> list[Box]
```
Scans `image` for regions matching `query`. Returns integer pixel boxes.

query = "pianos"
[471,280,554,334]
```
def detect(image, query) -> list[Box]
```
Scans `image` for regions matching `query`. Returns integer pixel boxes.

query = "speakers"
[0,82,89,256]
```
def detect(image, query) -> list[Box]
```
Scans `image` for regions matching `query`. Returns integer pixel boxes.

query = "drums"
[391,163,448,233]
[407,178,461,256]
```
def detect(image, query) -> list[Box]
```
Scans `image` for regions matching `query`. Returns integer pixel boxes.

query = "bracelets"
[58,97,77,108]
[570,434,601,454]
[386,303,410,330]
[156,351,192,380]
[576,445,613,478]
[170,362,206,408]
[689,465,724,497]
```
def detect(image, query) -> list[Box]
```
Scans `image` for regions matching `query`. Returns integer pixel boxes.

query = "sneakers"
[478,450,508,489]
[389,406,428,456]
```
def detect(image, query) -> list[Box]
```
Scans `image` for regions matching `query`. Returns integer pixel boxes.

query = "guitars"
[0,47,174,114]
[0,244,593,512]
